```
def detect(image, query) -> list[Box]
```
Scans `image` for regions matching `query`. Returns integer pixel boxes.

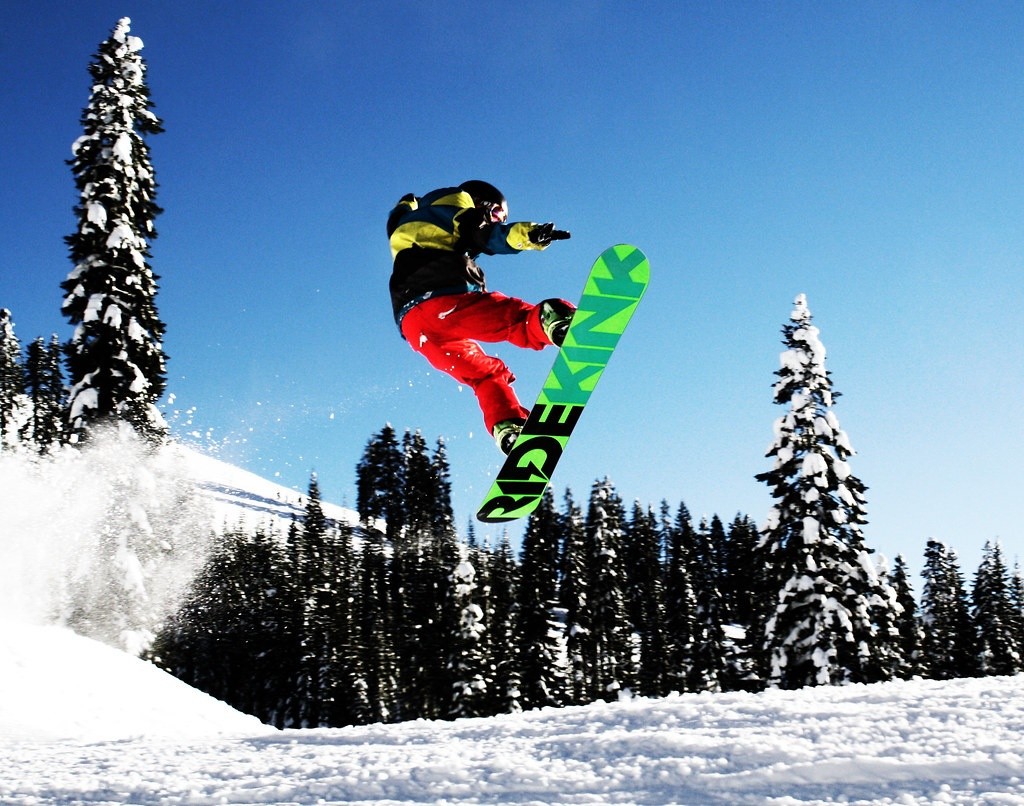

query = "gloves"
[529,223,571,246]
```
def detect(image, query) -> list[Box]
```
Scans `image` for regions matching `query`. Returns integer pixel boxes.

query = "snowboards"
[476,245,650,524]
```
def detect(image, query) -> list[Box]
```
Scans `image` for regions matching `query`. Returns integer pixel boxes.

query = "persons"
[387,180,578,455]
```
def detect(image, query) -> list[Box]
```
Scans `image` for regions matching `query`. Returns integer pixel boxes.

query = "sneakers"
[493,416,526,456]
[540,298,576,349]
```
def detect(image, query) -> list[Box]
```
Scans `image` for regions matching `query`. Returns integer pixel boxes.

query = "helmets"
[458,180,508,223]
